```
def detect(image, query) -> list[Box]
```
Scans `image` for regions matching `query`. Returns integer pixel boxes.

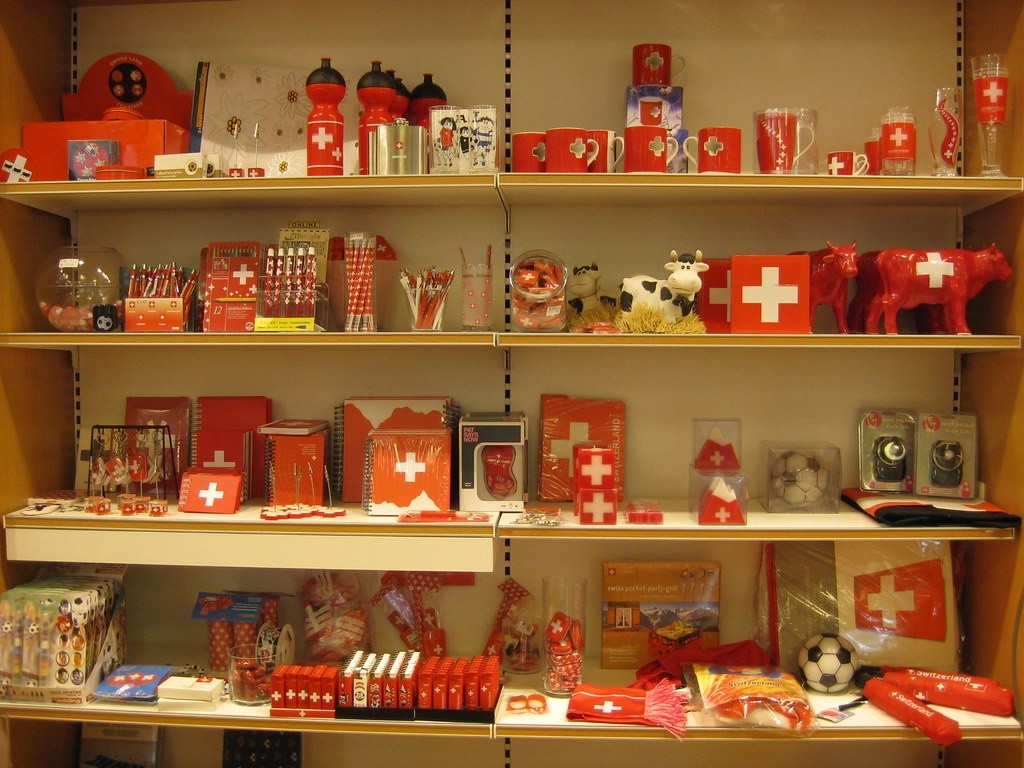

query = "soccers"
[770,449,830,509]
[799,633,858,693]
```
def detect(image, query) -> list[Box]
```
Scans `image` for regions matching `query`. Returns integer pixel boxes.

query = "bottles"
[34,246,129,333]
[306,58,348,176]
[356,60,447,175]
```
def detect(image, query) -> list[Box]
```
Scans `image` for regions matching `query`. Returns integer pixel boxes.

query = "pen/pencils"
[399,266,455,329]
[128,261,200,308]
[261,246,317,317]
[483,243,492,325]
[341,235,377,333]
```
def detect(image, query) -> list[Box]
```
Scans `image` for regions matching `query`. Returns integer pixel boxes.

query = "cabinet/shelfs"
[0,0,1024,768]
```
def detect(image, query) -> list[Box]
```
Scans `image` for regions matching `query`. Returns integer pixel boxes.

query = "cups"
[546,127,599,173]
[927,88,962,177]
[227,644,283,707]
[683,126,741,175]
[461,264,492,332]
[501,617,542,674]
[402,287,448,332]
[511,131,546,172]
[586,129,625,173]
[970,53,1009,177]
[756,111,815,173]
[625,125,678,174]
[631,43,688,87]
[467,105,501,175]
[542,574,588,699]
[429,105,461,174]
[325,260,400,331]
[639,97,670,127]
[827,104,917,176]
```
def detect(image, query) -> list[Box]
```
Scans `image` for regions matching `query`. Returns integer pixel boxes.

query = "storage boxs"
[20,120,191,179]
[626,86,688,174]
[601,560,721,669]
[125,297,194,332]
[157,676,225,713]
[154,153,222,179]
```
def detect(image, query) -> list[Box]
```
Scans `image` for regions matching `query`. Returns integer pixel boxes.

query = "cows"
[848,242,1013,339]
[620,249,710,324]
[567,262,622,314]
[789,240,859,334]
